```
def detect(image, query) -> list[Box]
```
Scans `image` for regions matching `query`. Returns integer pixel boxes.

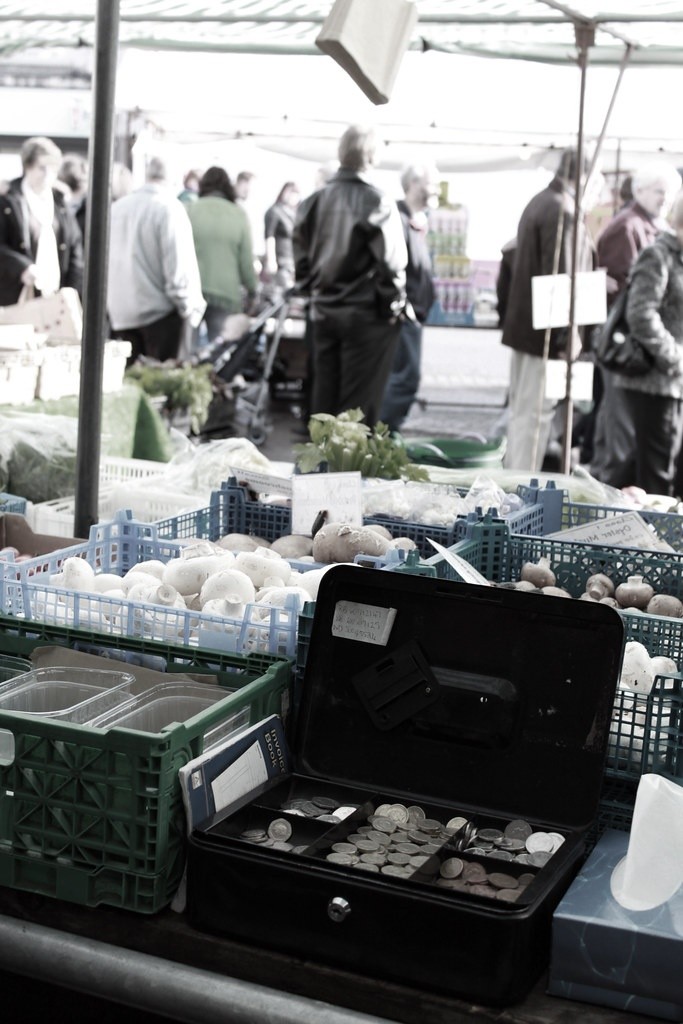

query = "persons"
[292,123,437,444]
[497,145,683,497]
[0,136,301,371]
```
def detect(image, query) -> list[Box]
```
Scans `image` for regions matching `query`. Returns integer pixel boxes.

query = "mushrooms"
[511,557,682,695]
[0,519,417,658]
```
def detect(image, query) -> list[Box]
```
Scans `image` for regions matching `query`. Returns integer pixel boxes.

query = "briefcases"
[183,565,629,1009]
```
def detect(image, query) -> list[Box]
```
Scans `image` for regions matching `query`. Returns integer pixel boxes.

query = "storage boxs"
[0,308,682,1023]
[423,212,478,326]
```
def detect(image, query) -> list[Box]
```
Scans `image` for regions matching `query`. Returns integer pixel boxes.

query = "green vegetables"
[290,407,432,485]
